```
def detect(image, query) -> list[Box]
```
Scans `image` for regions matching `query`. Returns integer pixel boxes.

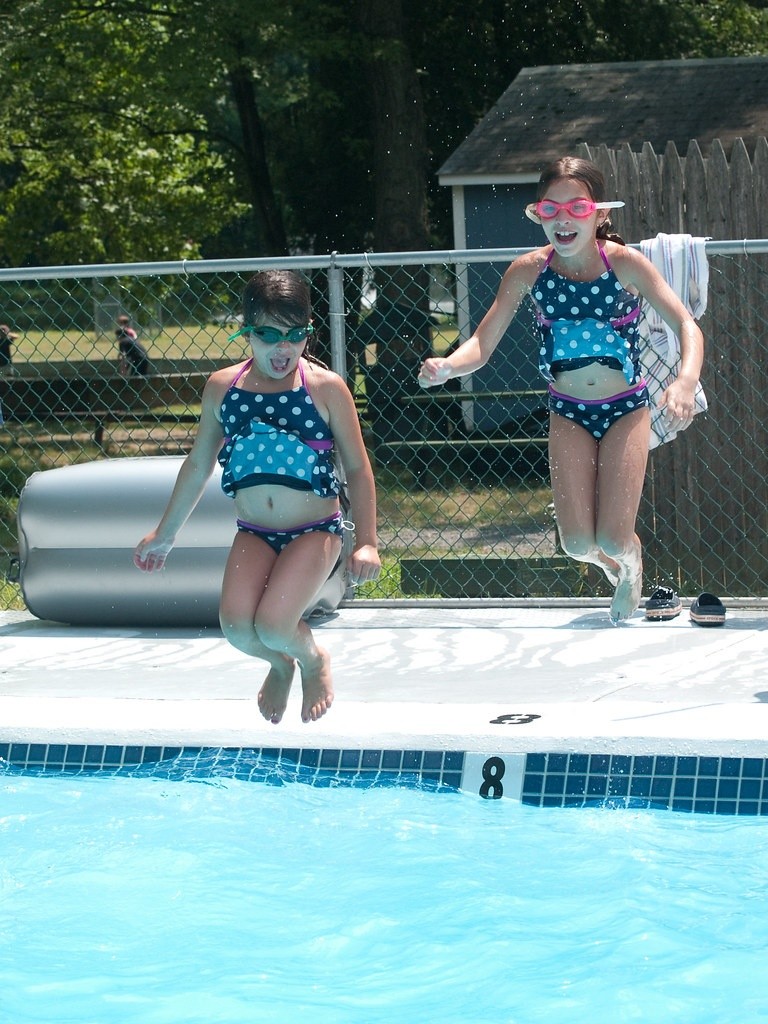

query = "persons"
[417,156,704,628]
[118,316,139,375]
[134,268,383,727]
[0,325,19,364]
[114,328,149,376]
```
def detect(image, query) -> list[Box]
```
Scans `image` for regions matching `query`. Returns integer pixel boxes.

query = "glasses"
[526,196,626,224]
[228,323,315,344]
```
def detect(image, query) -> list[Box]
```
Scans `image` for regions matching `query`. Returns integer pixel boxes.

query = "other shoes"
[689,592,725,626]
[645,586,682,620]
